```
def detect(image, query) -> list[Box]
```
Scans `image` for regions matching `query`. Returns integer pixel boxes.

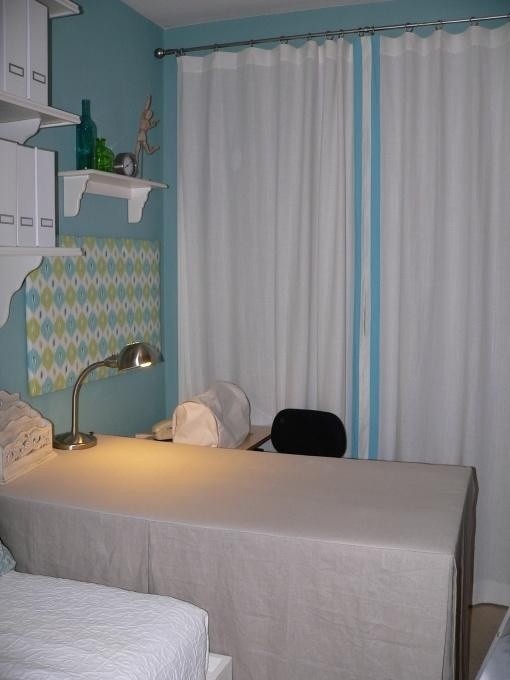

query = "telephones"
[153,419,173,440]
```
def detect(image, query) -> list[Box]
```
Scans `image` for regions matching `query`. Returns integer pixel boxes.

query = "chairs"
[271,409,347,458]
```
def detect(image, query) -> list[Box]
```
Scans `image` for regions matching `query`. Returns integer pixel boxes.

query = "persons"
[136,95,160,159]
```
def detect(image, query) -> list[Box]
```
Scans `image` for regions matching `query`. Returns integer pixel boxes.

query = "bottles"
[76,99,97,170]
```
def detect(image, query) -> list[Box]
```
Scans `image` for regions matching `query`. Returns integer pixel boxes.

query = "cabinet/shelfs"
[0,0,83,329]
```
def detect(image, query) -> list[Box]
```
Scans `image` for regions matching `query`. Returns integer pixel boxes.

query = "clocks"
[115,153,137,177]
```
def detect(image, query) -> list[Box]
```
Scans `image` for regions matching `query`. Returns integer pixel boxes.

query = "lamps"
[52,342,165,450]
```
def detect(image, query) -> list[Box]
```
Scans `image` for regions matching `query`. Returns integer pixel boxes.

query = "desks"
[122,424,271,451]
[1,430,479,680]
